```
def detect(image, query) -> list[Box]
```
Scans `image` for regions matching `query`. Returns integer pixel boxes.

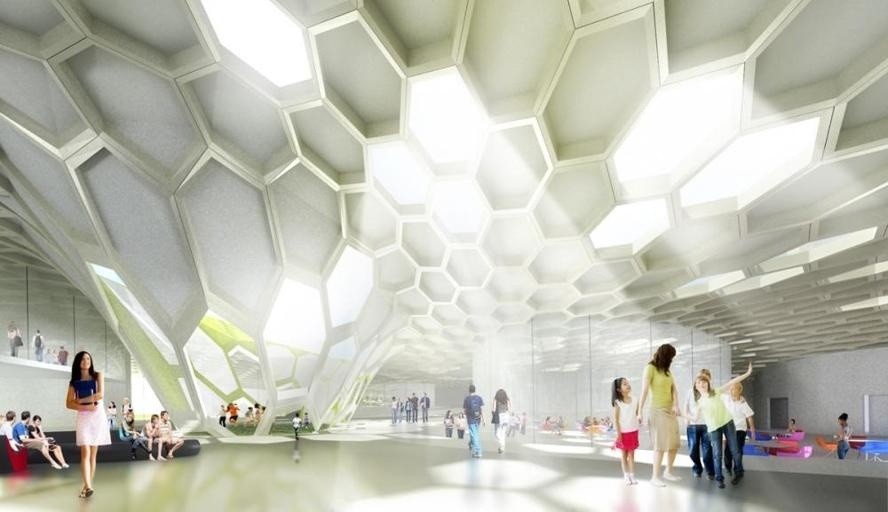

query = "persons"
[303,412,309,428]
[0,320,185,499]
[545,344,755,489]
[292,413,302,440]
[391,392,430,424]
[835,413,851,459]
[787,418,797,432]
[218,402,266,427]
[443,384,528,459]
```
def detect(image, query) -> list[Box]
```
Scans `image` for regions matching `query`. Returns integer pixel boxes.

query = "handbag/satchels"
[491,412,499,424]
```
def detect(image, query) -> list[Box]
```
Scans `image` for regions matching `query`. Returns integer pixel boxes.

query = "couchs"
[0,429,201,473]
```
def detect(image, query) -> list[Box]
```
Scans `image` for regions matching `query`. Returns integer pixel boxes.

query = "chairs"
[745,431,887,464]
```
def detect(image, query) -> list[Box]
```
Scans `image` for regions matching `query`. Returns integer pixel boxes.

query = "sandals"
[79,485,94,498]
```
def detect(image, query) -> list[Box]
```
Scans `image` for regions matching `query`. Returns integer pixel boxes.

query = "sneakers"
[623,469,744,488]
